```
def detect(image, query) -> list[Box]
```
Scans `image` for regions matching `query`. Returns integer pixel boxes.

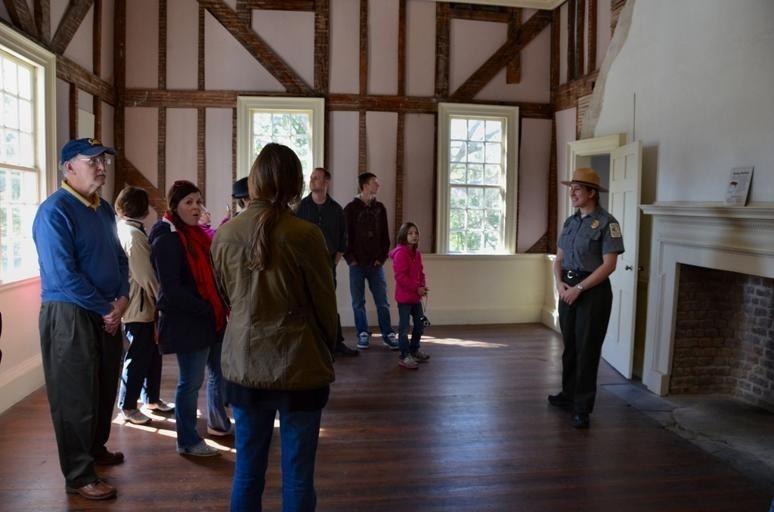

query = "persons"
[115,188,176,425]
[31,138,130,501]
[211,142,339,511]
[231,176,249,218]
[148,180,236,457]
[340,173,400,350]
[294,167,360,358]
[389,223,430,370]
[547,167,624,430]
[199,204,231,242]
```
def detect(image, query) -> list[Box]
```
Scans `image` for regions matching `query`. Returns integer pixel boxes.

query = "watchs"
[577,284,584,291]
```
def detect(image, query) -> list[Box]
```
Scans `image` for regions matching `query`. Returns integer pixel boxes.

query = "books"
[725,167,754,206]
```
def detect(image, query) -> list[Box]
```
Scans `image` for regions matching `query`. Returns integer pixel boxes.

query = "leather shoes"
[101,452,125,466]
[66,480,118,500]
[570,411,590,428]
[336,343,360,358]
[548,393,568,407]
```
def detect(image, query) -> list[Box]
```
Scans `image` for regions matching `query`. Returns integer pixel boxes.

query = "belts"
[560,270,589,279]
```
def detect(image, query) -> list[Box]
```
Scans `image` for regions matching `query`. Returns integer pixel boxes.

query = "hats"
[561,168,611,195]
[232,177,250,198]
[61,137,118,158]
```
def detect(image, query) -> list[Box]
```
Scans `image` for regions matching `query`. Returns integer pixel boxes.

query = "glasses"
[72,152,111,168]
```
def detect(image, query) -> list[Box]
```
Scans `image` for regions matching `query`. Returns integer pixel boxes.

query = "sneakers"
[207,427,238,437]
[121,409,153,426]
[357,332,371,348]
[146,399,177,414]
[411,352,430,361]
[177,440,221,456]
[382,333,400,348]
[399,353,418,368]
